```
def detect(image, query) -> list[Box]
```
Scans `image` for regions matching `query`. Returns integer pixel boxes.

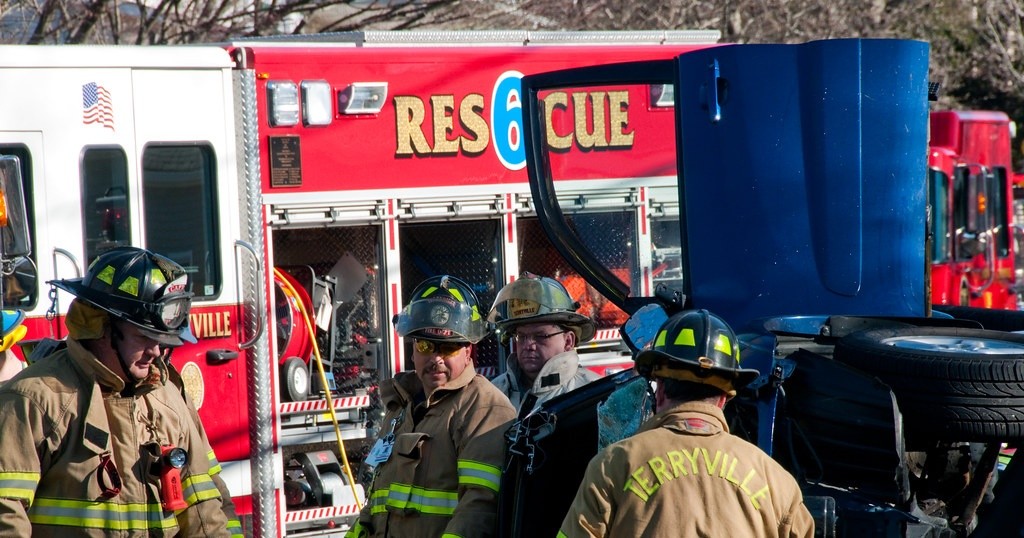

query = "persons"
[554,310,815,538]
[343,276,518,538]
[488,271,604,423]
[0,246,245,538]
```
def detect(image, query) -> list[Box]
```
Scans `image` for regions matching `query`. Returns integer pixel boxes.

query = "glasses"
[510,325,568,345]
[148,291,195,332]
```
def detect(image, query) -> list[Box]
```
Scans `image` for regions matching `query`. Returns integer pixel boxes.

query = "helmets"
[491,275,595,346]
[634,307,760,387]
[46,245,198,345]
[399,274,487,343]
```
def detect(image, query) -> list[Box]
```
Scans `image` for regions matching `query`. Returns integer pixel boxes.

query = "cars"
[491,33,1024,537]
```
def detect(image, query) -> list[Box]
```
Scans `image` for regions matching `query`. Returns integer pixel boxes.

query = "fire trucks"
[926,105,1024,324]
[0,26,747,538]
